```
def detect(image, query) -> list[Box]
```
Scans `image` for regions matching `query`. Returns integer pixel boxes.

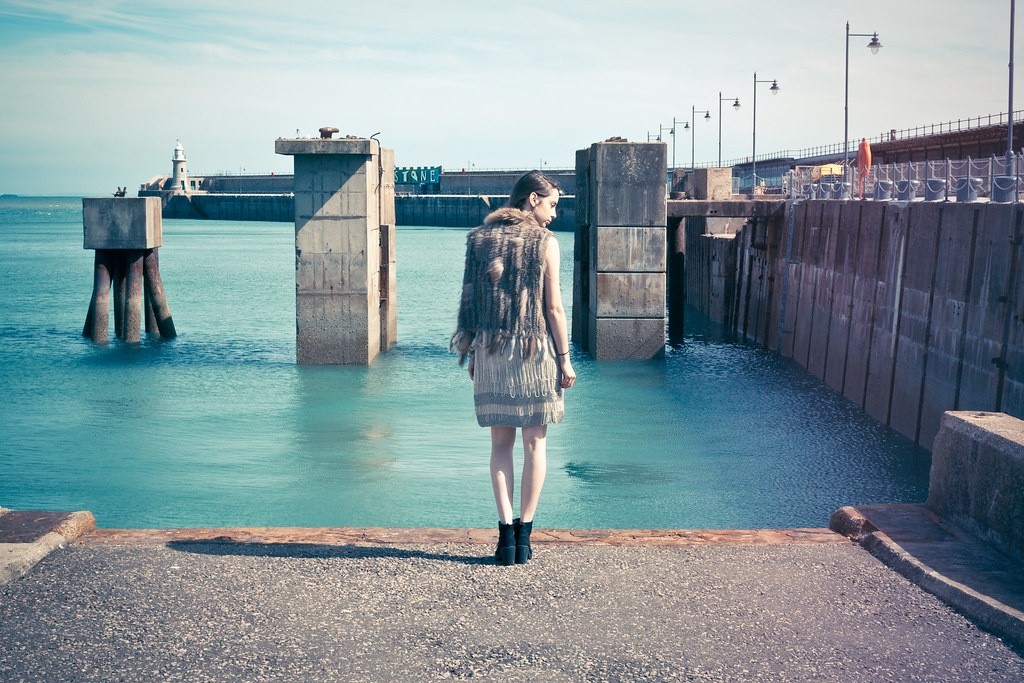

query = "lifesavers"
[857,141,872,174]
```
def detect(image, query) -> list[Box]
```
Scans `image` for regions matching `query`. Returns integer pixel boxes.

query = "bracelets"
[558,349,570,356]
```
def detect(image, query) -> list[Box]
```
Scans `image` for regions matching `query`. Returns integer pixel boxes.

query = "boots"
[495,521,516,565]
[515,517,534,564]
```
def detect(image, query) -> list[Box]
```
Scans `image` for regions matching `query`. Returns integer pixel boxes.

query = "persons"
[455,167,578,567]
[114,187,123,197]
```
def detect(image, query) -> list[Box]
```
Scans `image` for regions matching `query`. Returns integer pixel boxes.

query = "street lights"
[468,160,476,194]
[718,92,742,167]
[843,20,883,182]
[648,131,660,144]
[673,117,691,168]
[691,105,712,169]
[540,159,548,173]
[660,124,675,143]
[752,71,781,194]
[239,165,247,196]
